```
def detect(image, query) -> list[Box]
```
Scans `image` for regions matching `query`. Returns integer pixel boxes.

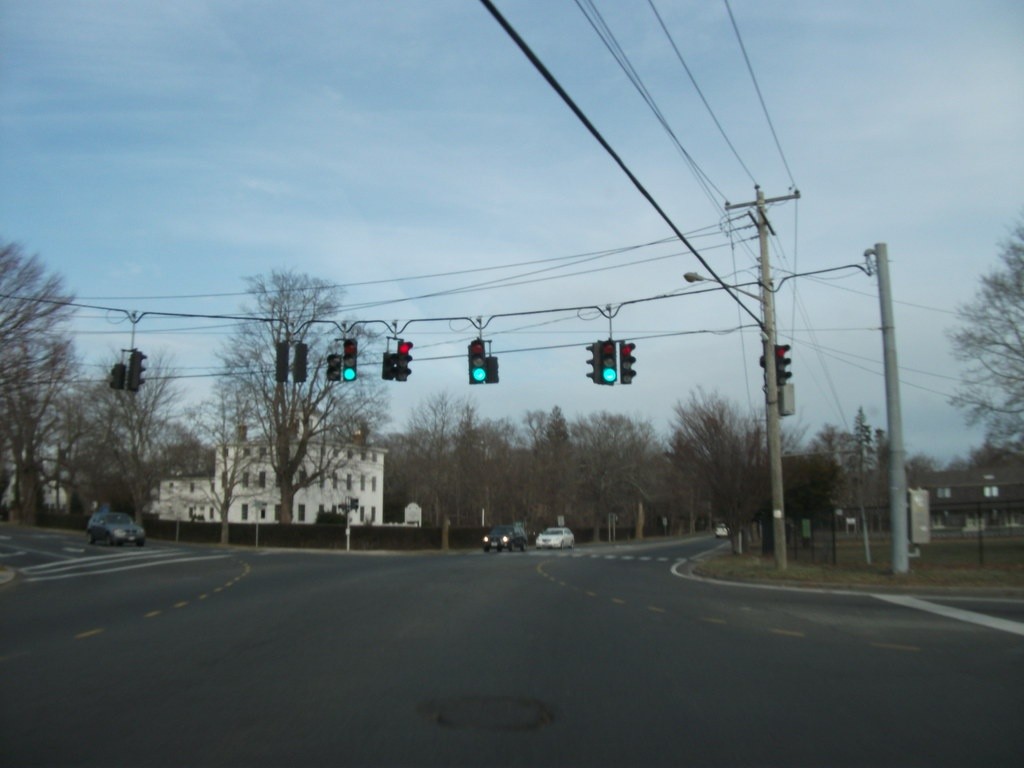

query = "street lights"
[684,271,787,571]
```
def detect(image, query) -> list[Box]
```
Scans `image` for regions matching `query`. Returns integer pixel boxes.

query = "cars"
[716,522,730,537]
[87,512,146,546]
[536,527,575,550]
[483,523,528,553]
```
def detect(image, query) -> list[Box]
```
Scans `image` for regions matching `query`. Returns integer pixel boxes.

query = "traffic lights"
[596,340,617,385]
[775,344,792,384]
[470,341,487,382]
[382,353,398,381]
[621,343,636,384]
[327,354,341,380]
[398,341,414,381]
[343,339,357,382]
[127,351,148,393]
